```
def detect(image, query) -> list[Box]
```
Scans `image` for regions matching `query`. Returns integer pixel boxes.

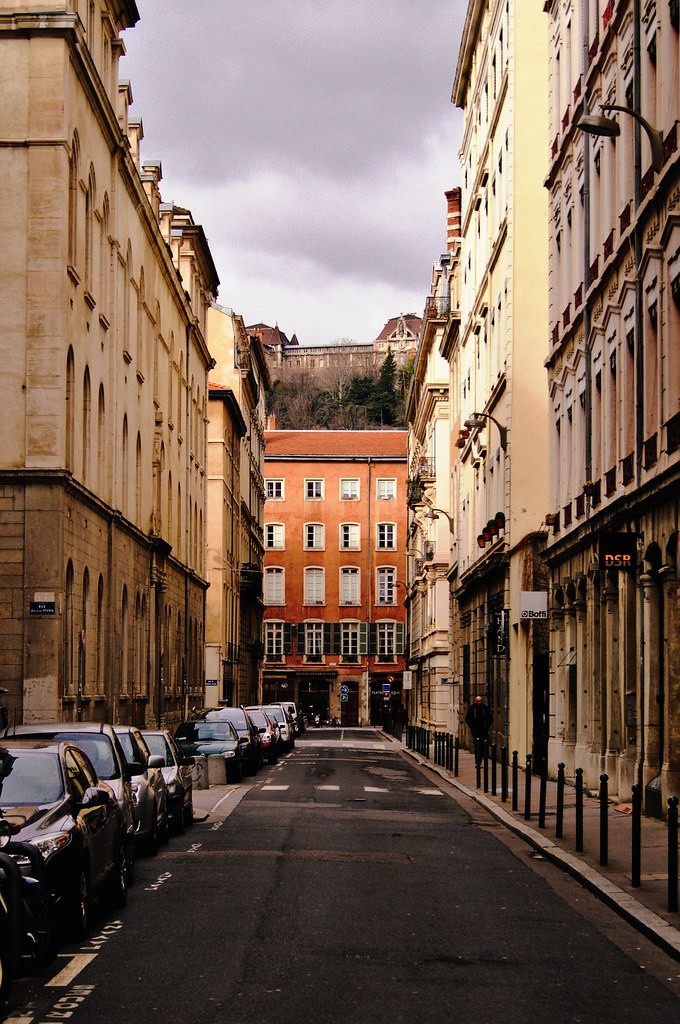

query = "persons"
[465,696,492,769]
[397,704,409,732]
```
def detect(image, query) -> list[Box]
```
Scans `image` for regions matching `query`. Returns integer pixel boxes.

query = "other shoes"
[475,764,484,769]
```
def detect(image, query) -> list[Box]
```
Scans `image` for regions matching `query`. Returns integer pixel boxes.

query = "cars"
[188,705,267,776]
[246,698,301,758]
[134,727,196,835]
[174,717,241,783]
[0,719,130,854]
[105,724,170,858]
[0,735,129,930]
